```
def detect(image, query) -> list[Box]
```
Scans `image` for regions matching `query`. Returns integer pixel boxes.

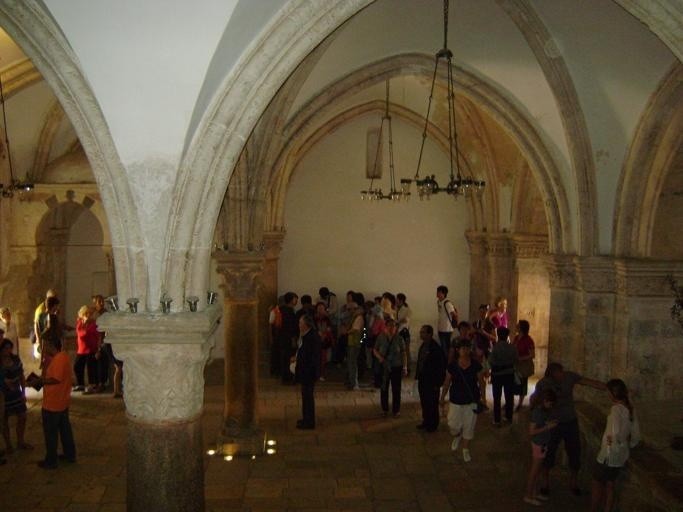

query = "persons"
[271,287,339,383]
[290,315,322,430]
[374,319,408,418]
[522,387,560,505]
[0,304,19,359]
[474,335,489,411]
[437,285,458,361]
[339,290,369,390]
[0,326,9,467]
[448,321,473,366]
[530,363,607,497]
[591,378,641,510]
[488,325,519,426]
[26,284,123,400]
[27,330,78,470]
[488,295,511,344]
[502,320,535,411]
[366,292,411,389]
[416,325,446,433]
[470,304,498,383]
[440,337,487,462]
[0,340,35,455]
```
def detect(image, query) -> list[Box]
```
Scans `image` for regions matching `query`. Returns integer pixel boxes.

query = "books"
[24,372,44,391]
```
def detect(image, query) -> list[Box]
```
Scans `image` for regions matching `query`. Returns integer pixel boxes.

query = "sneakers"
[451,437,461,449]
[38,461,57,469]
[570,486,583,496]
[57,454,76,463]
[415,421,440,433]
[462,448,472,463]
[523,487,551,507]
[296,418,316,430]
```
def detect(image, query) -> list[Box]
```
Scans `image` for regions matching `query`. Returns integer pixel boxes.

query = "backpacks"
[444,300,458,329]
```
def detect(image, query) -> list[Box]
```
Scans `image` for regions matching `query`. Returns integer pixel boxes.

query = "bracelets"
[403,367,407,369]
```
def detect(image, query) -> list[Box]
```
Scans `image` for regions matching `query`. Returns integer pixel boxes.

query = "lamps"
[354,71,412,204]
[393,1,489,201]
